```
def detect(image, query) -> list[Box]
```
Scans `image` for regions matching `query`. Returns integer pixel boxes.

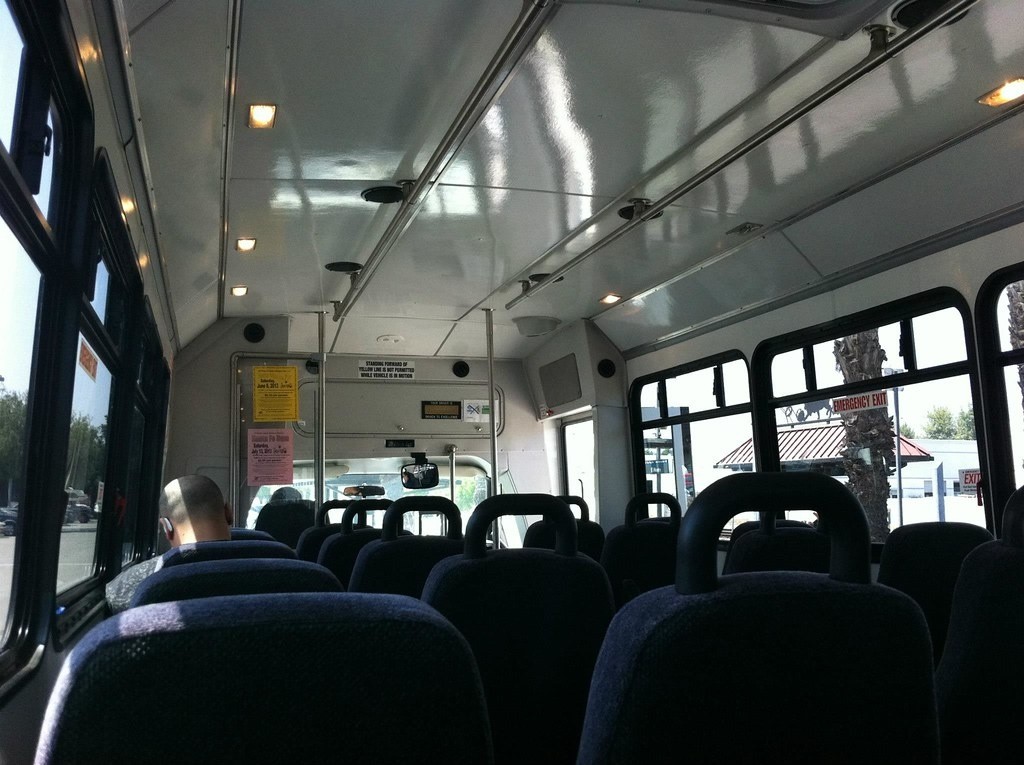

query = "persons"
[344,487,368,496]
[270,487,302,504]
[105,472,235,617]
[402,470,420,488]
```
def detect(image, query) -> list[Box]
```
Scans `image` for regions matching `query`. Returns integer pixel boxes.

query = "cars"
[64,500,93,523]
[0,504,19,537]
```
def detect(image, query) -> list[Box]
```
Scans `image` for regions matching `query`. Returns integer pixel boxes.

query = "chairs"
[721,509,831,576]
[523,496,605,564]
[420,494,616,765]
[878,522,997,678]
[347,496,466,600]
[128,558,347,608]
[32,593,499,765]
[722,519,812,576]
[231,530,279,541]
[575,473,940,765]
[295,499,374,564]
[934,483,1024,765]
[318,499,415,592]
[598,492,681,615]
[255,499,330,549]
[154,540,300,574]
